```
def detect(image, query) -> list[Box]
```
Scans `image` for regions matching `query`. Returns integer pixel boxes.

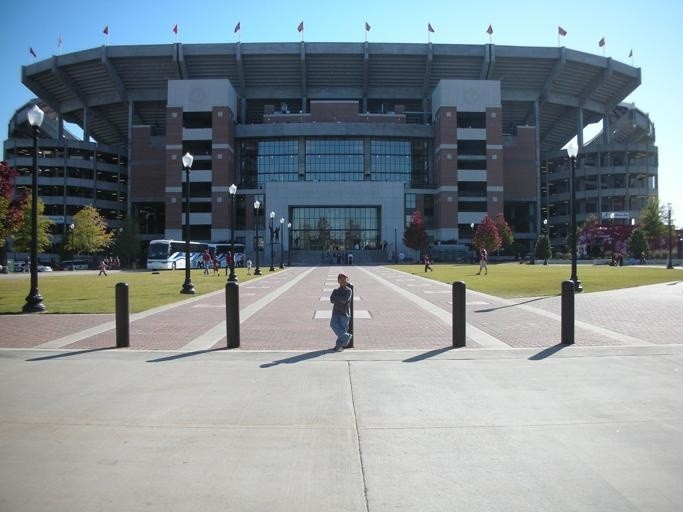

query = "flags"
[57,37,62,48]
[628,51,632,58]
[103,25,108,35]
[233,21,240,33]
[30,48,35,58]
[599,38,604,47]
[297,21,304,32]
[365,22,370,32]
[173,24,177,34]
[558,28,567,36]
[427,22,434,33]
[486,25,492,35]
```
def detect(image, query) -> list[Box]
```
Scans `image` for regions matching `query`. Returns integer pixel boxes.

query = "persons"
[613,248,624,266]
[22,256,30,274]
[114,256,120,268]
[109,256,112,269]
[423,256,432,272]
[97,260,107,276]
[202,249,244,276]
[475,245,487,275]
[246,256,252,275]
[330,273,352,351]
[377,239,405,264]
[326,242,364,265]
[103,256,108,269]
[640,250,647,265]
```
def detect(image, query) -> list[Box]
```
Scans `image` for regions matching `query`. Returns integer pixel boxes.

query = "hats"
[339,274,347,278]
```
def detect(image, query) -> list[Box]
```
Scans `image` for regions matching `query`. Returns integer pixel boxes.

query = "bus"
[146,239,209,271]
[60,260,88,270]
[206,243,246,269]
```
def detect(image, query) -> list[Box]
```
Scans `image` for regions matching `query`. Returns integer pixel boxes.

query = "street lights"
[253,201,262,275]
[666,202,674,269]
[470,222,475,264]
[268,211,292,272]
[22,104,45,314]
[70,223,76,271]
[543,219,548,265]
[227,183,239,282]
[394,226,398,264]
[180,152,196,294]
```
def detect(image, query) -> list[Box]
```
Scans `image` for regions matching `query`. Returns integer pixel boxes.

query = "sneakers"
[341,334,352,347]
[327,346,342,352]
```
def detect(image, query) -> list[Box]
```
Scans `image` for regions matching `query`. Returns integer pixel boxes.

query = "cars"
[0,263,52,273]
